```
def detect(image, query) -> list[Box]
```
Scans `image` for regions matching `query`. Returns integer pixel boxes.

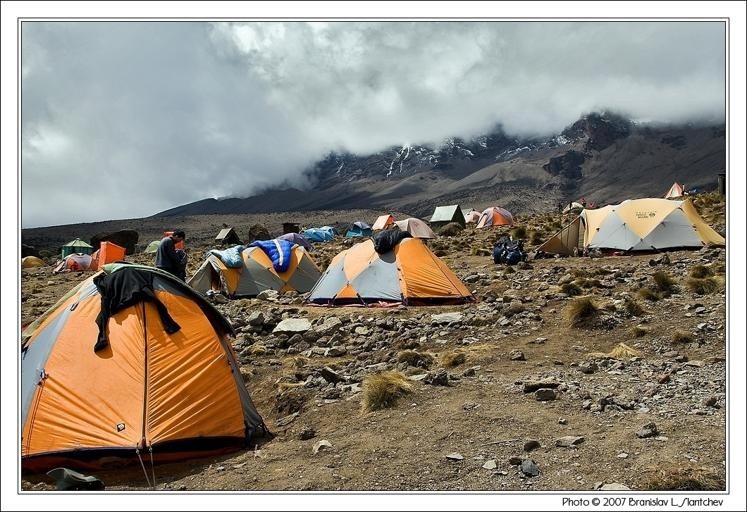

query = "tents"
[536,198,725,255]
[666,182,689,198]
[187,241,324,300]
[21,263,276,473]
[21,231,185,269]
[562,202,584,215]
[275,205,516,303]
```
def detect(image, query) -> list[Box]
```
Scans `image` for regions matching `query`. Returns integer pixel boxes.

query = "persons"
[558,203,563,213]
[681,184,685,196]
[581,199,586,207]
